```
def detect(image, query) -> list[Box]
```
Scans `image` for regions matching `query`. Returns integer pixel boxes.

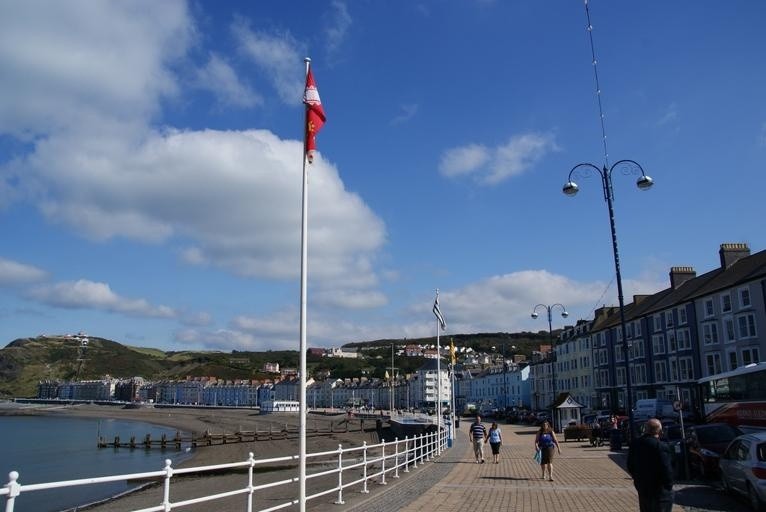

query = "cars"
[481,407,550,426]
[582,398,674,424]
[658,424,766,512]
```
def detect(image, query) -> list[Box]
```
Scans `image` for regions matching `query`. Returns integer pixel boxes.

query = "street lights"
[454,349,470,422]
[491,345,517,421]
[563,159,654,446]
[531,303,568,403]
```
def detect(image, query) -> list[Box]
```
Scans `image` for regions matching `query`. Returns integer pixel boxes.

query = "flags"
[433,294,446,332]
[450,340,459,365]
[302,61,327,163]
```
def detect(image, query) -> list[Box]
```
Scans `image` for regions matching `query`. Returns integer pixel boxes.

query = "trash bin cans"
[609,429,622,451]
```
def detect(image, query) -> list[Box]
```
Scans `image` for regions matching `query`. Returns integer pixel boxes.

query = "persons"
[535,419,561,482]
[610,416,619,430]
[468,416,487,464]
[627,418,674,512]
[486,422,502,460]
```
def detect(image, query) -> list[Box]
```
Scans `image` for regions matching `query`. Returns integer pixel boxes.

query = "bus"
[698,361,766,425]
